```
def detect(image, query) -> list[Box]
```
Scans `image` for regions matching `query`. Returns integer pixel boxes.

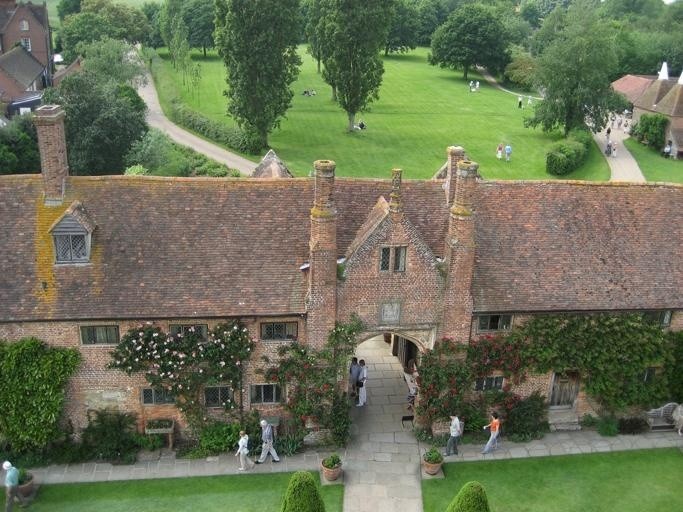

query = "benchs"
[645,402,683,430]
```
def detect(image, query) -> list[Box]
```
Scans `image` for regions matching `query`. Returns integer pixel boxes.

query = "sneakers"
[239,468,246,470]
[442,451,450,456]
[272,459,279,462]
[255,461,263,463]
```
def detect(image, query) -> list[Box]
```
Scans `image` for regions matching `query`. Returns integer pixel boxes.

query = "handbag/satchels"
[357,382,363,387]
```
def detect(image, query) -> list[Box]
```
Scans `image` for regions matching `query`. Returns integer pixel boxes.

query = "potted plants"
[143,419,176,435]
[320,455,343,481]
[17,467,34,496]
[423,449,443,475]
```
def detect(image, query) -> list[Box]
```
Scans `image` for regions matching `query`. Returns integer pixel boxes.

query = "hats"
[3,461,11,470]
[261,420,267,426]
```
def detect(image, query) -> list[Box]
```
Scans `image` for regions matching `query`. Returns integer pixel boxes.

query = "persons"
[528,96,532,105]
[518,95,522,108]
[358,120,366,128]
[255,420,280,464]
[3,461,28,512]
[663,144,670,158]
[585,110,628,158]
[470,80,480,91]
[350,358,360,397]
[442,414,461,456]
[480,413,500,454]
[356,360,367,406]
[505,144,511,161]
[304,88,318,96]
[497,143,503,160]
[235,431,255,470]
